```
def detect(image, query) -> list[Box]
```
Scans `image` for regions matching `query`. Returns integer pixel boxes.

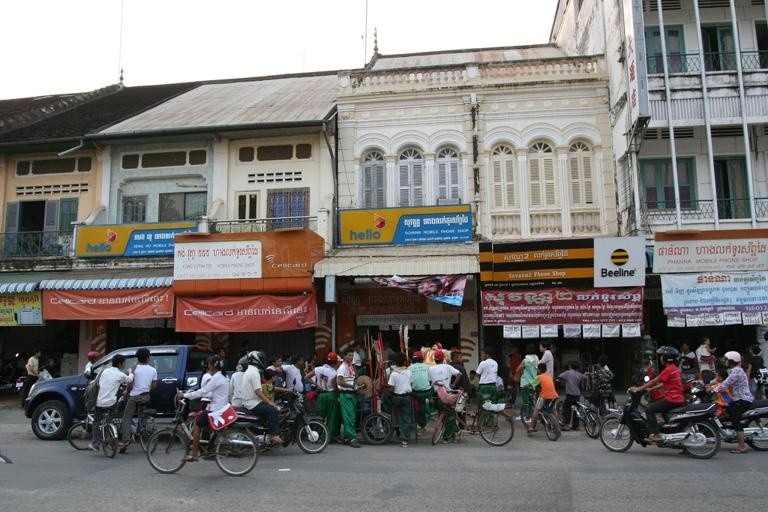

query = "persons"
[19,347,158,454]
[417,277,443,297]
[177,340,618,462]
[627,332,767,455]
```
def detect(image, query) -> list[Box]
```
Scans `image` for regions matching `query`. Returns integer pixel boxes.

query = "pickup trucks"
[22,345,287,442]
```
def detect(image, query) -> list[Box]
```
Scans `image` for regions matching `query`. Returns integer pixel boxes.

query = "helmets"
[724,351,741,362]
[88,352,102,363]
[248,351,267,370]
[236,356,248,372]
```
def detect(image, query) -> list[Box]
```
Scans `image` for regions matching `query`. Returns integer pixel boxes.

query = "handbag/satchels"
[84,381,98,414]
[207,404,238,431]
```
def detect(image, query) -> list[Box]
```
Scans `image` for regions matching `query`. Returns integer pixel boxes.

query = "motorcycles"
[223,378,332,455]
[14,363,57,397]
[598,381,768,460]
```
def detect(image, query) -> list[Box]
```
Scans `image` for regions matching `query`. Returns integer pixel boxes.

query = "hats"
[434,351,445,361]
[656,346,680,362]
[412,351,423,359]
[327,353,341,362]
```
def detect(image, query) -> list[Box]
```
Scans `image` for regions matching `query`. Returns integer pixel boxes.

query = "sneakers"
[453,430,463,441]
[352,439,362,448]
[440,439,448,444]
[527,428,536,433]
[644,435,658,441]
[730,446,748,453]
[401,441,409,448]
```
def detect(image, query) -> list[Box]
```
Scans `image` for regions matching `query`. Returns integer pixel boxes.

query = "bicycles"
[144,391,260,477]
[598,385,615,419]
[429,380,516,447]
[66,403,120,458]
[520,383,561,441]
[121,382,160,454]
[553,376,602,439]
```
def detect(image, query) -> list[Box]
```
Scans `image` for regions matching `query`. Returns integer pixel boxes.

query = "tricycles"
[353,395,394,446]
